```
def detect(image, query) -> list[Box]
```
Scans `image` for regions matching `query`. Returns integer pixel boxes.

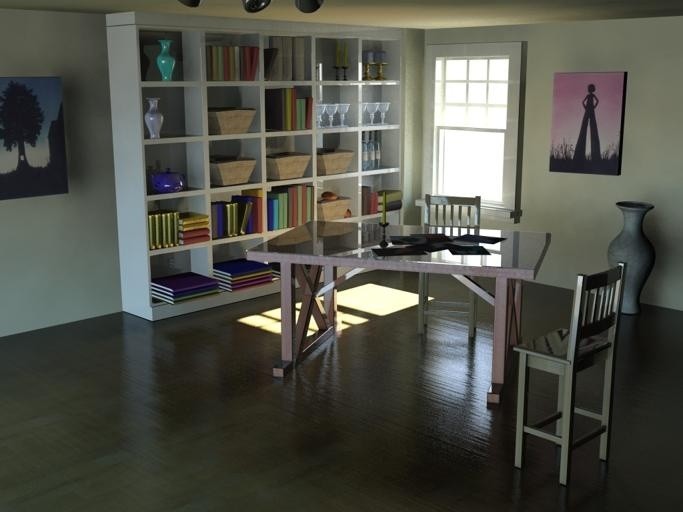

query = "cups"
[326,105,337,128]
[379,103,390,125]
[367,103,379,126]
[362,103,367,112]
[337,104,350,127]
[316,105,326,129]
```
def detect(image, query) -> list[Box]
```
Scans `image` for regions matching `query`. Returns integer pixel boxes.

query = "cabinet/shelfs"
[105,11,403,322]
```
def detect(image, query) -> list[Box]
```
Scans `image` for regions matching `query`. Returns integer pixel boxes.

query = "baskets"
[208,106,257,135]
[266,151,311,180]
[318,196,352,221]
[317,147,355,176]
[210,155,257,186]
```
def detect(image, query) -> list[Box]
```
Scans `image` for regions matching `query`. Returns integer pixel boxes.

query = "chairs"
[512,261,628,486]
[417,194,481,338]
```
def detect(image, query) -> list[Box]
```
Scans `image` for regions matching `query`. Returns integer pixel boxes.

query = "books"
[150,208,210,249]
[267,87,313,129]
[210,188,263,239]
[206,45,259,80]
[151,271,220,306]
[364,183,402,213]
[268,183,315,229]
[214,258,273,292]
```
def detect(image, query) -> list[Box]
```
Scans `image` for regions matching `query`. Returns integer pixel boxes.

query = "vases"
[145,97,163,138]
[607,201,655,314]
[158,39,175,82]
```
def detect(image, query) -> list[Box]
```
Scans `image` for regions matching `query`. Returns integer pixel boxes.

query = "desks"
[246,221,552,403]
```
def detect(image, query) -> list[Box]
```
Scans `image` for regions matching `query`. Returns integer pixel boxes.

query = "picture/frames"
[0,76,68,201]
[549,71,627,176]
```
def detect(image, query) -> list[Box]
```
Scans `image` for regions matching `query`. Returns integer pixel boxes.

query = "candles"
[383,191,387,225]
